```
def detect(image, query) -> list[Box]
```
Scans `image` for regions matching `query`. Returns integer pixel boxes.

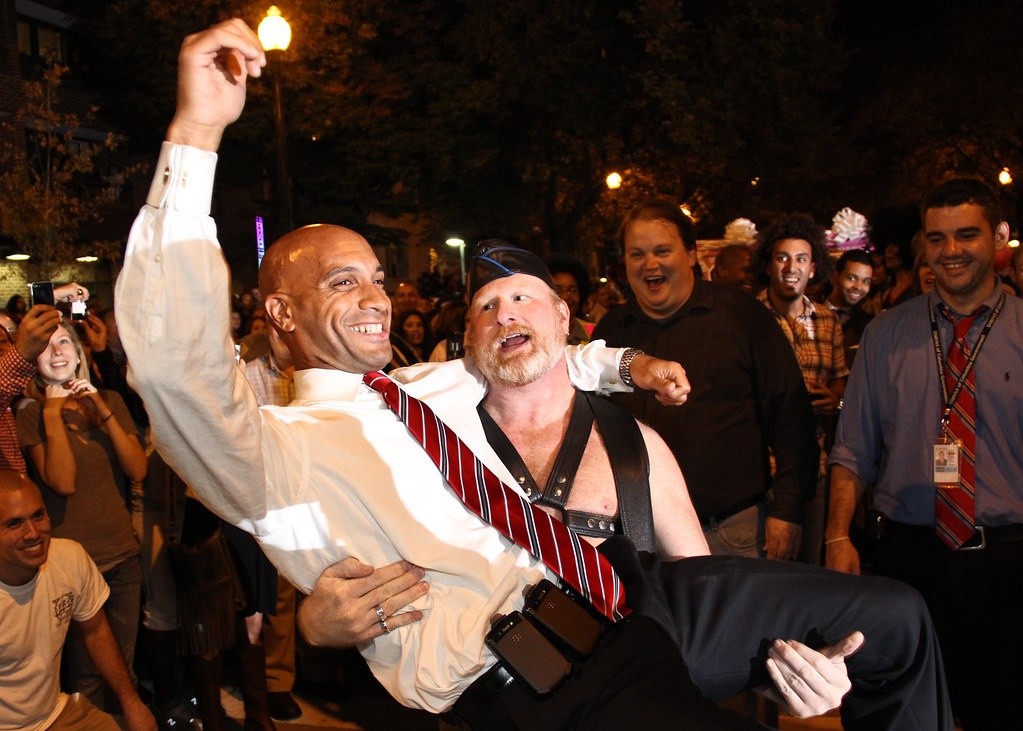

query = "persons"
[935,450,946,466]
[113,19,960,731]
[0,184,1023,731]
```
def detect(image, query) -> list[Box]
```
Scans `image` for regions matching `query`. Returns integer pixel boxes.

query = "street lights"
[605,171,623,259]
[255,4,294,228]
[446,234,467,285]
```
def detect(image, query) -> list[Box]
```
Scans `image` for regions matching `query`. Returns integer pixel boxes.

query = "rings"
[376,621,392,636]
[77,289,83,296]
[373,606,387,622]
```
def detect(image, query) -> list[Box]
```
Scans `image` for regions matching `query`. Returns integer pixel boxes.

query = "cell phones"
[71,300,87,321]
[523,580,599,653]
[485,610,571,698]
[30,281,55,309]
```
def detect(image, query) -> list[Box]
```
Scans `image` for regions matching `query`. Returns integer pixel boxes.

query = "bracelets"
[823,535,850,545]
[619,348,647,389]
[830,391,844,417]
[100,411,113,422]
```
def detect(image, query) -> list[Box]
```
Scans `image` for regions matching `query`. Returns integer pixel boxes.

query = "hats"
[466,238,557,308]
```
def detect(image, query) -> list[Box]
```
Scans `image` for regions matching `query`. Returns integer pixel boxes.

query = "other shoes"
[91,619,356,731]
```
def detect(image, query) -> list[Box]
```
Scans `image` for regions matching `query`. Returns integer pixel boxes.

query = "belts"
[450,586,578,728]
[865,509,1023,552]
[699,491,766,531]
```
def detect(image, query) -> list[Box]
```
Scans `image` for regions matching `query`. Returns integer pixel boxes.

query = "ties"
[934,304,990,552]
[366,371,632,623]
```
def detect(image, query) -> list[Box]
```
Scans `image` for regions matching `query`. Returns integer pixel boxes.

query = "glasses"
[555,285,580,298]
